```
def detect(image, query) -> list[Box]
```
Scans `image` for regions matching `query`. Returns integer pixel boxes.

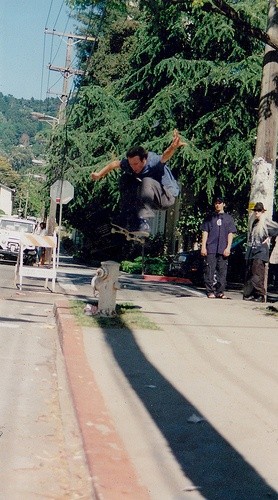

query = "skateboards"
[110,222,150,244]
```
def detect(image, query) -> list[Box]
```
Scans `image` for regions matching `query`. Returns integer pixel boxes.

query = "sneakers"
[139,204,155,219]
[133,221,151,234]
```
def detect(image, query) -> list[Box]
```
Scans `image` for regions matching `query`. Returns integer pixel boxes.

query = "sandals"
[207,292,228,299]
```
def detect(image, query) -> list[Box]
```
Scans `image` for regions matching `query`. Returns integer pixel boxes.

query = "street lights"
[29,111,65,264]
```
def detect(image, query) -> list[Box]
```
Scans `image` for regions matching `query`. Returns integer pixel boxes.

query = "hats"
[214,197,224,203]
[250,203,267,211]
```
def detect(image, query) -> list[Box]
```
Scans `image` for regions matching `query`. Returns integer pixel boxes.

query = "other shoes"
[36,262,44,264]
[244,296,265,302]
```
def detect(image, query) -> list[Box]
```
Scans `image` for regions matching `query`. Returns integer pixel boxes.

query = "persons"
[200,197,237,298]
[243,202,278,301]
[91,129,188,232]
[34,222,46,264]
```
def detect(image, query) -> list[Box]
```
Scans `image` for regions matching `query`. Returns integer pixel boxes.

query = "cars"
[169,233,250,285]
[0,216,40,266]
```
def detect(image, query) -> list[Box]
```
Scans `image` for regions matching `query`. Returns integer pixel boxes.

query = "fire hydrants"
[90,260,123,317]
